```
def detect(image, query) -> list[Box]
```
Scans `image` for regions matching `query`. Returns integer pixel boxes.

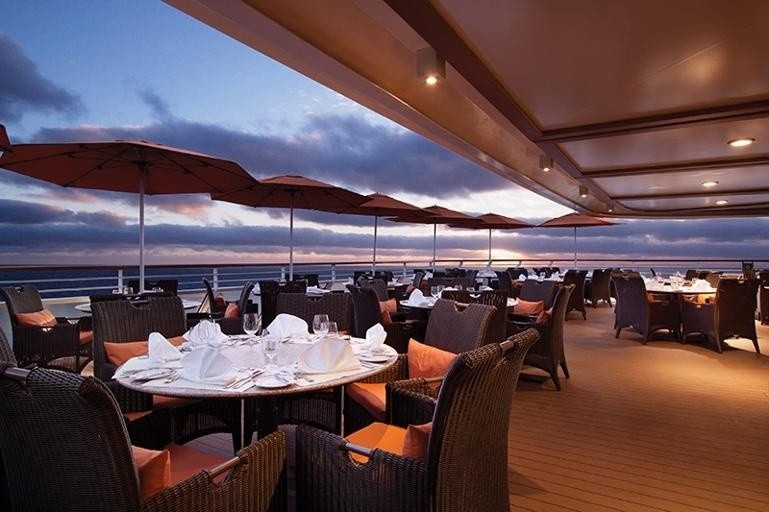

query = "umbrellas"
[536,212,621,268]
[338,192,442,275]
[383,204,484,273]
[210,173,374,281]
[448,213,536,265]
[0,139,260,292]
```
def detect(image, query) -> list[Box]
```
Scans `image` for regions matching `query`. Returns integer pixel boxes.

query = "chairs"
[611,269,769,354]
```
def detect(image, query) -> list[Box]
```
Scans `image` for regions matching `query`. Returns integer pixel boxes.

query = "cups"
[113,286,163,295]
[430,283,475,303]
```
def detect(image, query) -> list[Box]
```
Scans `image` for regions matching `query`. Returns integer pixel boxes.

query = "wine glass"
[655,271,685,291]
[539,271,546,281]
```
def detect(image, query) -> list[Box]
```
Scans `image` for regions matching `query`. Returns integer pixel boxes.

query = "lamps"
[537,154,554,173]
[579,184,588,199]
[606,201,616,213]
[415,49,448,86]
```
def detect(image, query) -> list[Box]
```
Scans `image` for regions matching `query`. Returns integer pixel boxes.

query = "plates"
[125,310,394,392]
